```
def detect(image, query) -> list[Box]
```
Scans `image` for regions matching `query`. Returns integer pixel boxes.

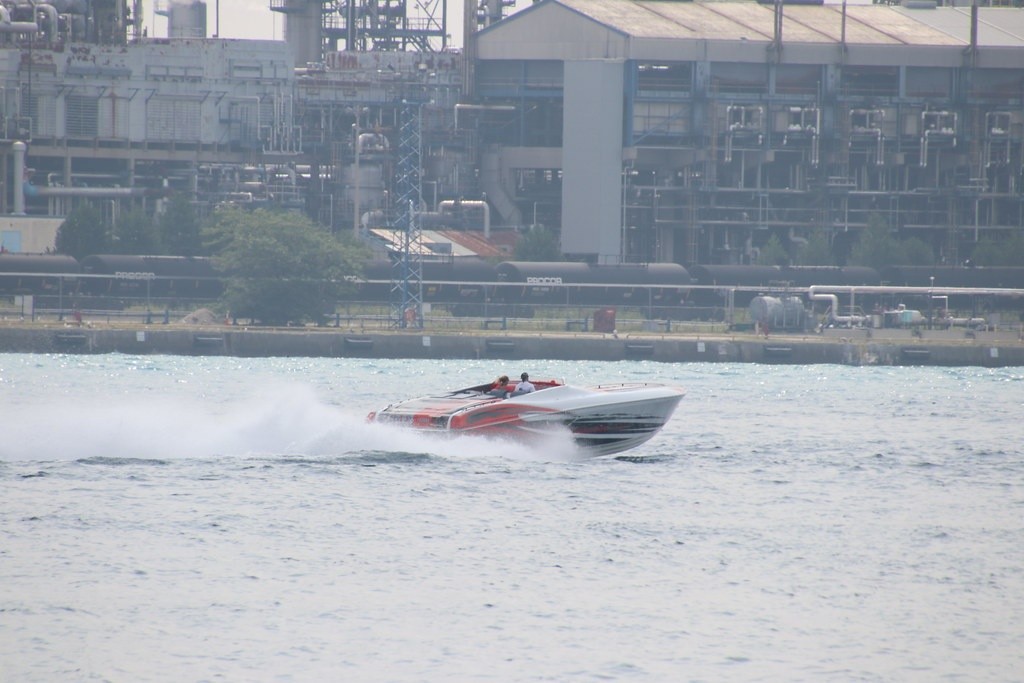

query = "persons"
[515,373,536,392]
[492,376,514,392]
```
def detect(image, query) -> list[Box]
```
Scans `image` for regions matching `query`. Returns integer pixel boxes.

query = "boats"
[363,377,688,460]
